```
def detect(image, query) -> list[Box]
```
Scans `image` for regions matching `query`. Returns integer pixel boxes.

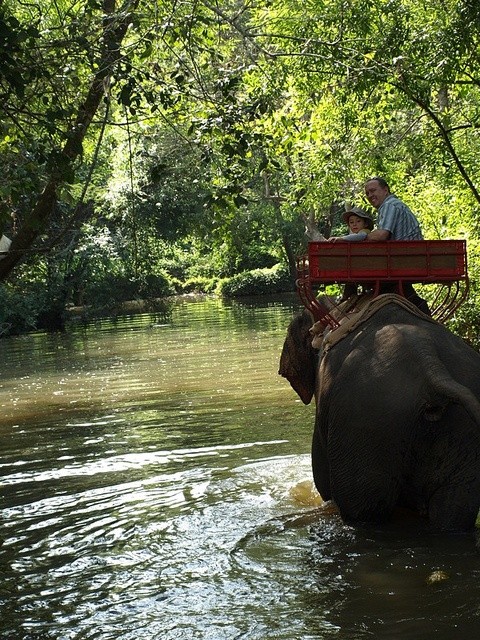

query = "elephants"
[278,291,480,533]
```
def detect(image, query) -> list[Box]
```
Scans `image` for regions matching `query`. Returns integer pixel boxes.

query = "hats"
[342,207,374,232]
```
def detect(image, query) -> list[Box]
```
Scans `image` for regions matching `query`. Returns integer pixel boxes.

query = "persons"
[364,177,424,241]
[326,205,374,243]
